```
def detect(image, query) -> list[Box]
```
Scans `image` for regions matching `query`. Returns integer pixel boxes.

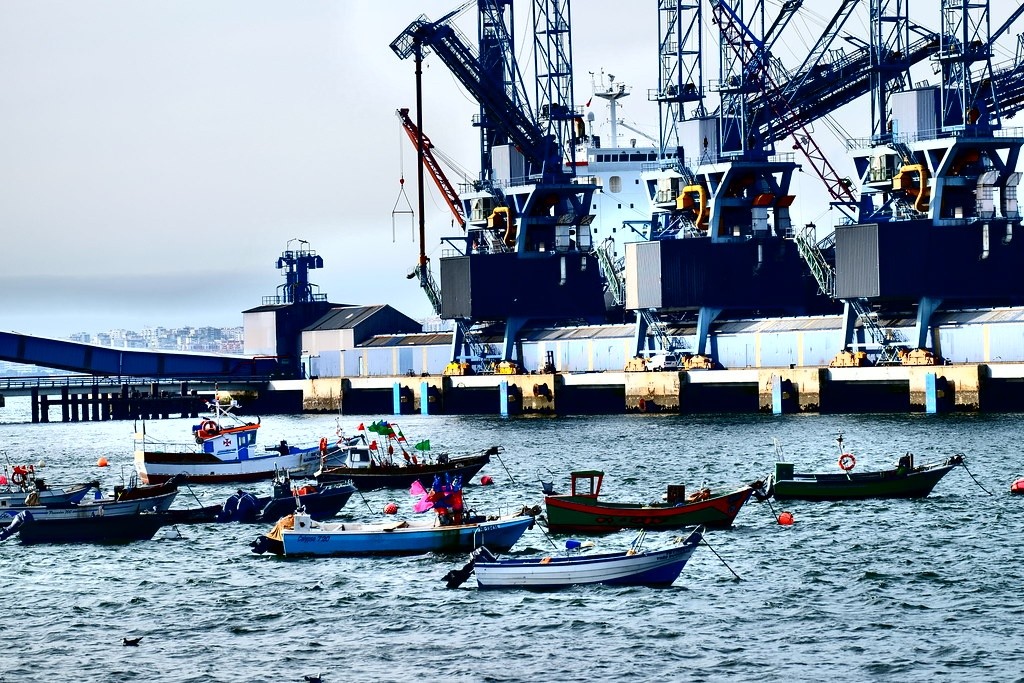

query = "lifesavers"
[12,472,26,485]
[202,420,218,436]
[686,490,711,504]
[320,438,327,463]
[839,454,856,471]
[639,399,647,413]
[302,485,317,494]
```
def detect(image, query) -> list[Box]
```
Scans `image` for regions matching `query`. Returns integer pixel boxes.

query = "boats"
[750,451,963,499]
[250,507,544,560]
[316,439,497,486]
[212,465,358,521]
[543,471,761,531]
[0,487,179,543]
[165,505,223,521]
[134,383,363,482]
[441,525,707,591]
[0,465,94,508]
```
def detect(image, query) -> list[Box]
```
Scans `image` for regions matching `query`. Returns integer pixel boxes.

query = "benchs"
[539,557,551,564]
[382,522,405,531]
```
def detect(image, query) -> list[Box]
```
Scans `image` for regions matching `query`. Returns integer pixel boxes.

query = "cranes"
[708,0,932,224]
[398,108,490,250]
